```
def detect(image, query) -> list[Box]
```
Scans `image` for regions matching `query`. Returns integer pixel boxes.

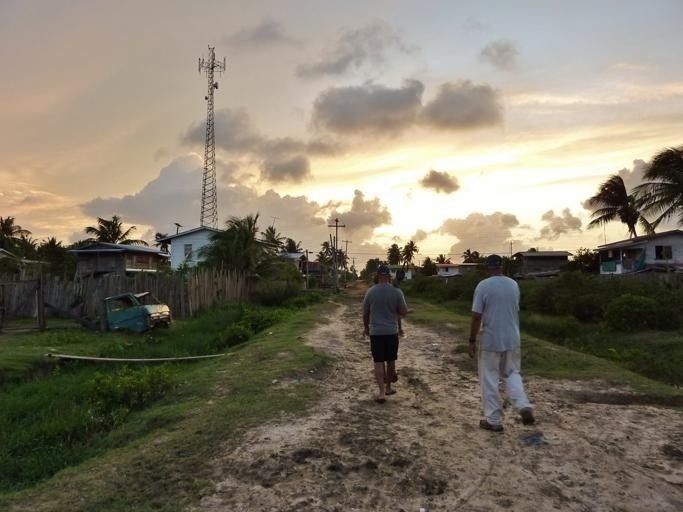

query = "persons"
[467,254,536,432]
[362,265,409,404]
[370,272,408,383]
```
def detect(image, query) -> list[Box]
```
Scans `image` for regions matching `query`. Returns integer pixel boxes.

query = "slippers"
[374,369,398,403]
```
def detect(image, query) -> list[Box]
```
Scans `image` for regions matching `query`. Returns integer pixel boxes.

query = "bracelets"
[469,339,476,343]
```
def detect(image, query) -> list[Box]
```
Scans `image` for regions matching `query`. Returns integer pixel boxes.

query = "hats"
[486,254,502,266]
[377,265,390,275]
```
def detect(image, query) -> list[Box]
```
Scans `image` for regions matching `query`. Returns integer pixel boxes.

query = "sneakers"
[521,408,535,425]
[480,420,502,431]
